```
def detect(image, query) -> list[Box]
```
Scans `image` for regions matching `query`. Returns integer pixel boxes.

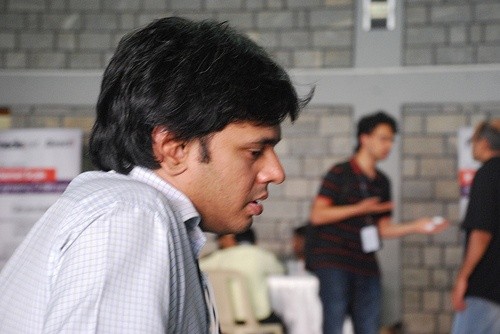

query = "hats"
[466,119,500,143]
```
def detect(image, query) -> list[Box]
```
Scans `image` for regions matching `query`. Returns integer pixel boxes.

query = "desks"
[267,274,355,334]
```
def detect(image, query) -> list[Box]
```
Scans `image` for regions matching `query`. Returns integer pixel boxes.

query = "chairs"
[209,269,283,334]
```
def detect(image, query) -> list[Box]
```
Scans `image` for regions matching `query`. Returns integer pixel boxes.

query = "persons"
[0,16,316,334]
[310,111,448,334]
[450,120,500,334]
[198,225,310,334]
